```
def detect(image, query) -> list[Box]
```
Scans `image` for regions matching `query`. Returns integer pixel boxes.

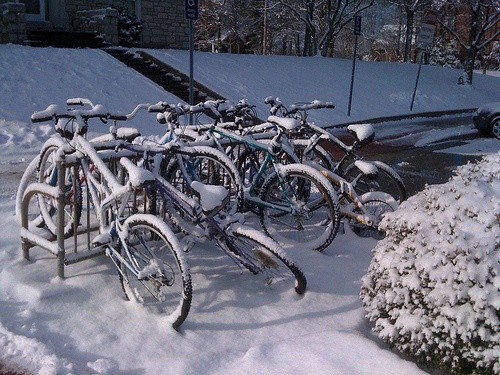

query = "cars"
[473,103,500,140]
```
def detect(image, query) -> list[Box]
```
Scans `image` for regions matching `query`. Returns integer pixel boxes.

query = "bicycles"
[30,92,427,331]
